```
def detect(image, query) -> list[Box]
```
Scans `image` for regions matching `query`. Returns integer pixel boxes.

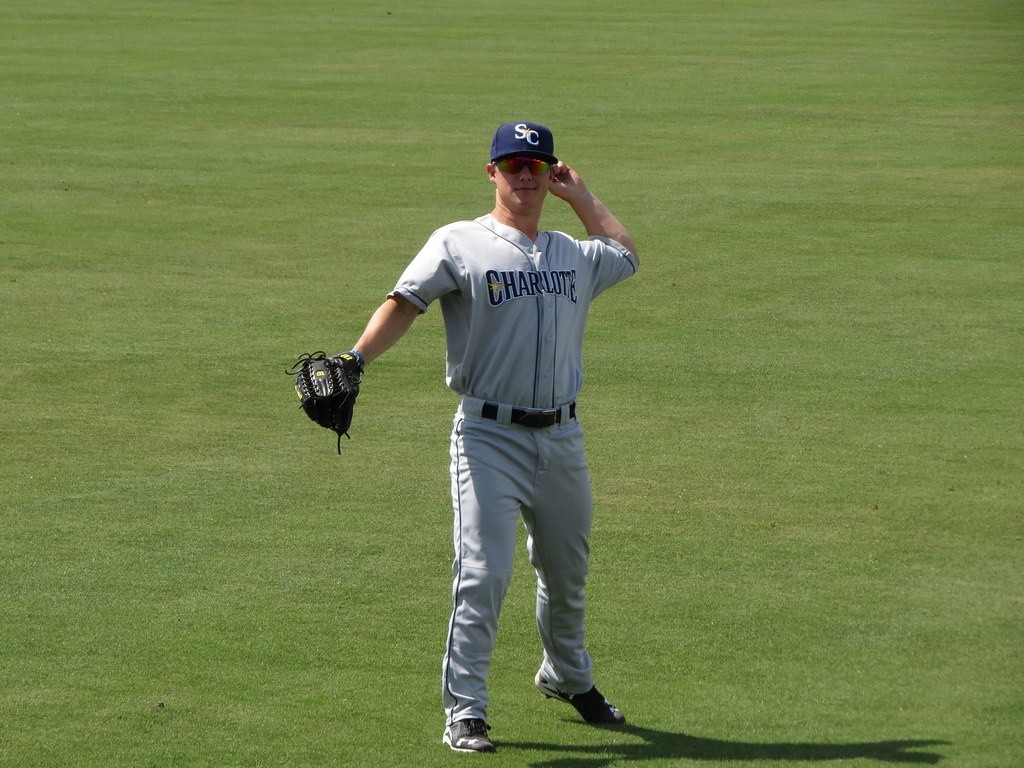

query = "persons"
[283,120,641,752]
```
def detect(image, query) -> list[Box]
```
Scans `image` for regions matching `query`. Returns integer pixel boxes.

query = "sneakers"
[442,718,494,753]
[535,668,625,724]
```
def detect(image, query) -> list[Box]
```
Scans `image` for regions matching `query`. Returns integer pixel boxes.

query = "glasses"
[492,154,555,176]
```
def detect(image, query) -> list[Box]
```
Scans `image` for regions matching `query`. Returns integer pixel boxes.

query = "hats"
[489,121,558,166]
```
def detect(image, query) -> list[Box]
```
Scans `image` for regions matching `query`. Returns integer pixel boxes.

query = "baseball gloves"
[294,351,364,437]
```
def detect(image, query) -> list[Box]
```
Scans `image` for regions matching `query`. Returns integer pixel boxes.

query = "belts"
[481,403,575,429]
